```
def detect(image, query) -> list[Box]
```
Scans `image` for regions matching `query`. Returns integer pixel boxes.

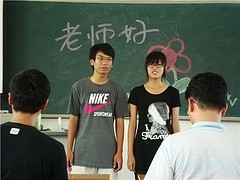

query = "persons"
[0,68,68,180]
[127,51,180,180]
[144,72,240,180]
[66,42,124,180]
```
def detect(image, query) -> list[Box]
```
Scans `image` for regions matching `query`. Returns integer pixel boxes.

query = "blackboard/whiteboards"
[0,0,240,123]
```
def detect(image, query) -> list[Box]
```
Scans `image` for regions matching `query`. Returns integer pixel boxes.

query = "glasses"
[146,63,165,68]
